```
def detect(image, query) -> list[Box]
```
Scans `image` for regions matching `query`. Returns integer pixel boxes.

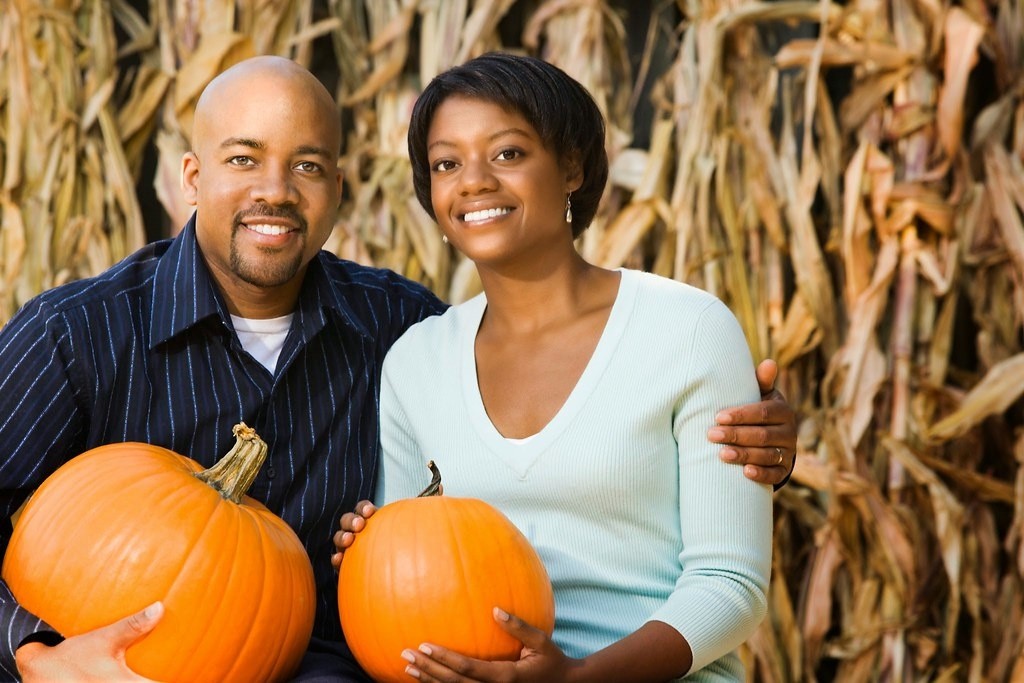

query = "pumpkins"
[338,460,556,683]
[2,421,318,683]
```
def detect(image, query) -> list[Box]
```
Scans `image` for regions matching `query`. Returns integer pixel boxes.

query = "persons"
[331,52,773,683]
[0,55,797,683]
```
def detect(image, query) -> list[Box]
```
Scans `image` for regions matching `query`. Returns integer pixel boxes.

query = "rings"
[776,448,783,464]
